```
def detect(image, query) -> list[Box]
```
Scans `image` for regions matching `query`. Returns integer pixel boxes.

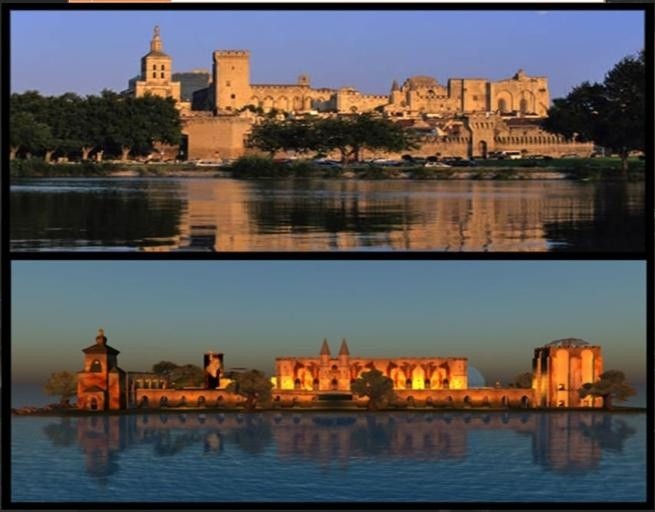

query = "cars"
[48,150,645,167]
